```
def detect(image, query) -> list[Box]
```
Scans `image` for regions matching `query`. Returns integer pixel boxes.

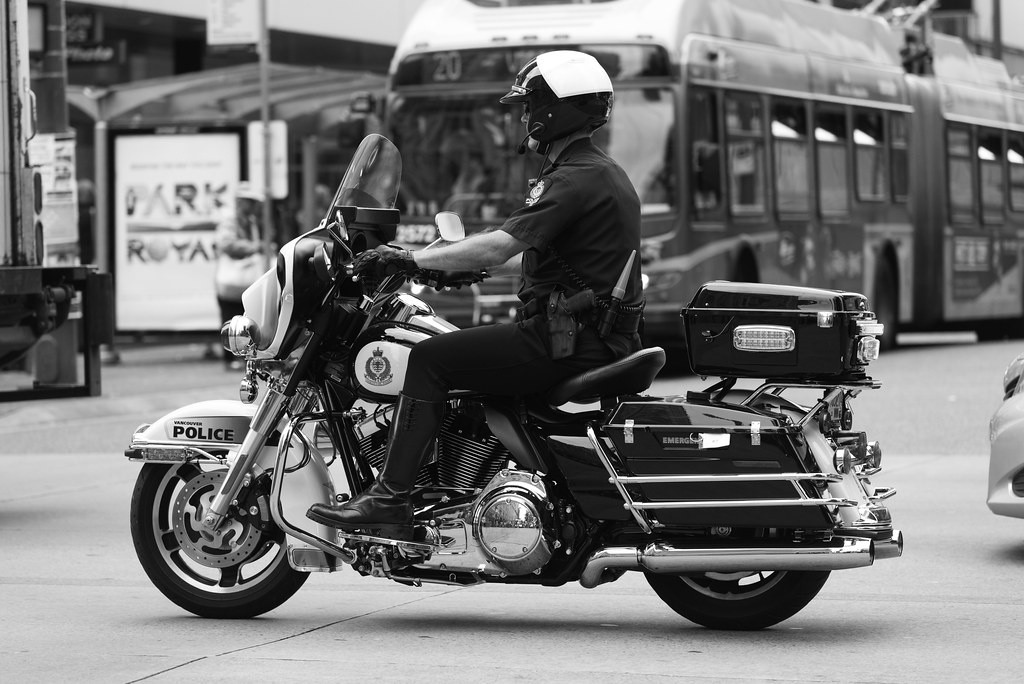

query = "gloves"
[353,246,420,279]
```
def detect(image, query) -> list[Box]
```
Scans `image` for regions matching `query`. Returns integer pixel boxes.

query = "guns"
[546,287,596,360]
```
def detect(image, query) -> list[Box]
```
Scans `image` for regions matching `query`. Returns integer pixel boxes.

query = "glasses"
[523,102,530,114]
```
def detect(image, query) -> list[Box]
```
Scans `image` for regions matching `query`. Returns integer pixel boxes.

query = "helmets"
[500,50,614,142]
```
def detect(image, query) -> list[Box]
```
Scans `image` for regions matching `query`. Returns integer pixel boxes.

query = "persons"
[307,50,644,539]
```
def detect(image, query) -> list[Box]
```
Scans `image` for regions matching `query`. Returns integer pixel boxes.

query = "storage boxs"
[601,401,834,544]
[681,281,884,384]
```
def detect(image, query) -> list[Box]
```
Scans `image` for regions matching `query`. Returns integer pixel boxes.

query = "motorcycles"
[121,133,905,632]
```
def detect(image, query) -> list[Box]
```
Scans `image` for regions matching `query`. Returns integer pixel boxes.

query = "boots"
[307,389,445,529]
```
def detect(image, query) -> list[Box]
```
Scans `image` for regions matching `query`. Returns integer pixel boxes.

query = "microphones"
[517,128,540,154]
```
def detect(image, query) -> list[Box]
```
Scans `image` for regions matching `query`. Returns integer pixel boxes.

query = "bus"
[347,0,1024,354]
